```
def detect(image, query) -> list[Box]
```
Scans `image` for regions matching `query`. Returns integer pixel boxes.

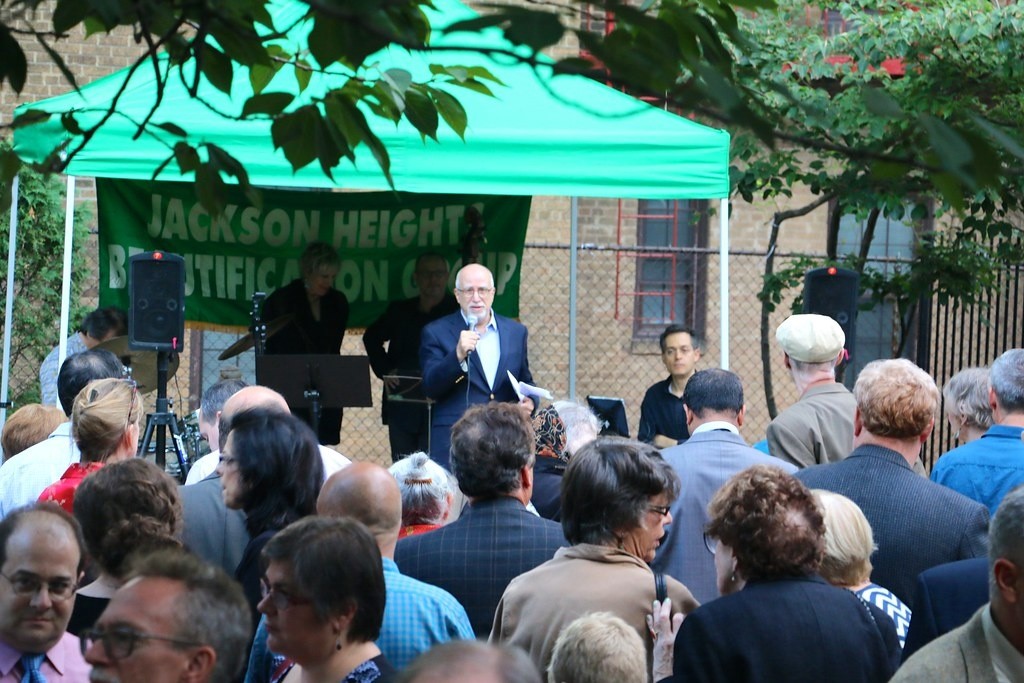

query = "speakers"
[803,267,857,367]
[128,252,185,352]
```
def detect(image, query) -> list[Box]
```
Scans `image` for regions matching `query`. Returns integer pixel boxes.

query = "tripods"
[136,351,193,484]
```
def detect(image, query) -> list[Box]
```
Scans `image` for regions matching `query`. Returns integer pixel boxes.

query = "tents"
[0,1,728,428]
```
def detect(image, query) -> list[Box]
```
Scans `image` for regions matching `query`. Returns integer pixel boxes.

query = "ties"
[21,652,46,683]
[308,295,321,323]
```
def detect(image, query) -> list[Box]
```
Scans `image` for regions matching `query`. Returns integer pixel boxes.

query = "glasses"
[1,571,78,602]
[256,572,313,612]
[218,453,237,463]
[120,377,137,423]
[647,504,670,516]
[78,627,199,659]
[702,530,722,554]
[458,287,494,298]
[417,269,444,277]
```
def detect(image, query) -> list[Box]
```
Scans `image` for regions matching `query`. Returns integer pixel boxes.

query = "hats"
[778,314,846,362]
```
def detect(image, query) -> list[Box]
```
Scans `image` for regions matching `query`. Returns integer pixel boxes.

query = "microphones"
[467,315,479,357]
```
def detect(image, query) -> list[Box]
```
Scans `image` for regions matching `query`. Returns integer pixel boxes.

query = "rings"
[651,628,660,639]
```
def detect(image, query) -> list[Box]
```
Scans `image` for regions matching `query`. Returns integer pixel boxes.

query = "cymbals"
[90,336,180,395]
[218,312,297,361]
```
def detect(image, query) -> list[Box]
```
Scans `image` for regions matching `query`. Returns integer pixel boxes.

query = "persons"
[0,502,93,683]
[244,461,476,683]
[257,515,394,683]
[638,324,700,449]
[396,640,542,683]
[548,612,648,683]
[887,487,1024,683]
[85,552,253,683]
[363,253,460,463]
[421,264,539,473]
[488,436,700,683]
[0,308,1024,662]
[647,464,902,683]
[262,244,349,444]
